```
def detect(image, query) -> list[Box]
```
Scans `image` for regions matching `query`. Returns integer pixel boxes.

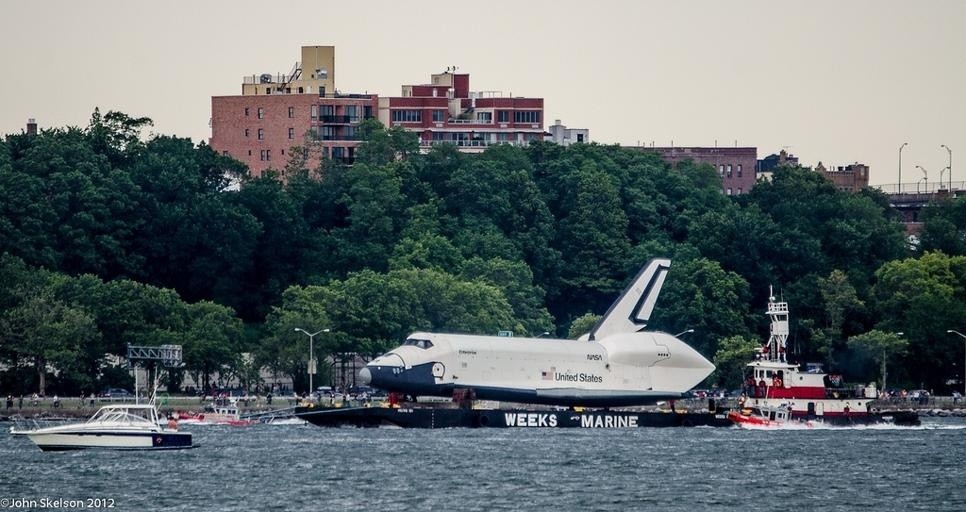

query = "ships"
[729,284,926,429]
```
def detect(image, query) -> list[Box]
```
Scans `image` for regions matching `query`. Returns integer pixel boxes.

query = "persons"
[53,394,59,409]
[184,379,291,407]
[32,392,38,405]
[737,374,783,415]
[953,390,962,405]
[876,386,935,408]
[830,374,844,386]
[777,346,787,363]
[166,417,177,429]
[693,383,730,399]
[90,392,96,407]
[80,390,85,405]
[756,343,771,361]
[301,378,369,406]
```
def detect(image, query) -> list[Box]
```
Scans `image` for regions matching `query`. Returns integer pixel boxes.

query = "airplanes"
[355,255,728,407]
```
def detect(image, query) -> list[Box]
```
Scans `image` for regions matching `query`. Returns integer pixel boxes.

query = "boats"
[290,403,739,429]
[159,401,255,427]
[7,402,203,451]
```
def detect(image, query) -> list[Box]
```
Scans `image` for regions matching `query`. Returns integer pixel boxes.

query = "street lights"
[536,330,551,338]
[939,143,953,194]
[916,165,928,194]
[898,142,909,194]
[917,176,928,194]
[882,331,906,388]
[294,327,332,394]
[676,329,695,338]
[946,329,966,339]
[940,166,950,188]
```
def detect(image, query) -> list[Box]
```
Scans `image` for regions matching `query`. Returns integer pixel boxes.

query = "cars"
[688,388,743,400]
[877,387,931,405]
[97,388,135,403]
[314,381,390,401]
